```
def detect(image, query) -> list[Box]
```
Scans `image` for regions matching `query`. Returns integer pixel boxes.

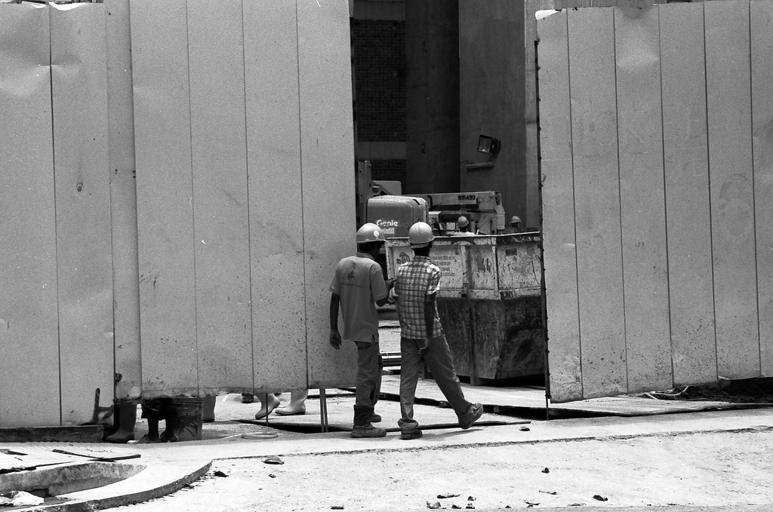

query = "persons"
[392,221,484,440]
[328,222,396,438]
[504,215,524,234]
[104,402,136,445]
[141,401,177,443]
[255,389,309,421]
[451,215,477,236]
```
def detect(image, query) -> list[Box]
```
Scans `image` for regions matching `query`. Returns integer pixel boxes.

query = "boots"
[105,403,136,443]
[275,389,307,415]
[350,404,386,437]
[255,393,280,419]
[203,396,216,422]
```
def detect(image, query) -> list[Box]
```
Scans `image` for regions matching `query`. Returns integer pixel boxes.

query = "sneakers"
[401,432,422,439]
[463,403,483,429]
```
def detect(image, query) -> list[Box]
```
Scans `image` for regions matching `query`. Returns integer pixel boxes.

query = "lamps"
[475,134,501,155]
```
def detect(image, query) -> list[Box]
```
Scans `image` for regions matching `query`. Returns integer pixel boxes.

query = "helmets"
[511,215,521,224]
[408,221,435,244]
[356,223,387,243]
[458,216,469,228]
